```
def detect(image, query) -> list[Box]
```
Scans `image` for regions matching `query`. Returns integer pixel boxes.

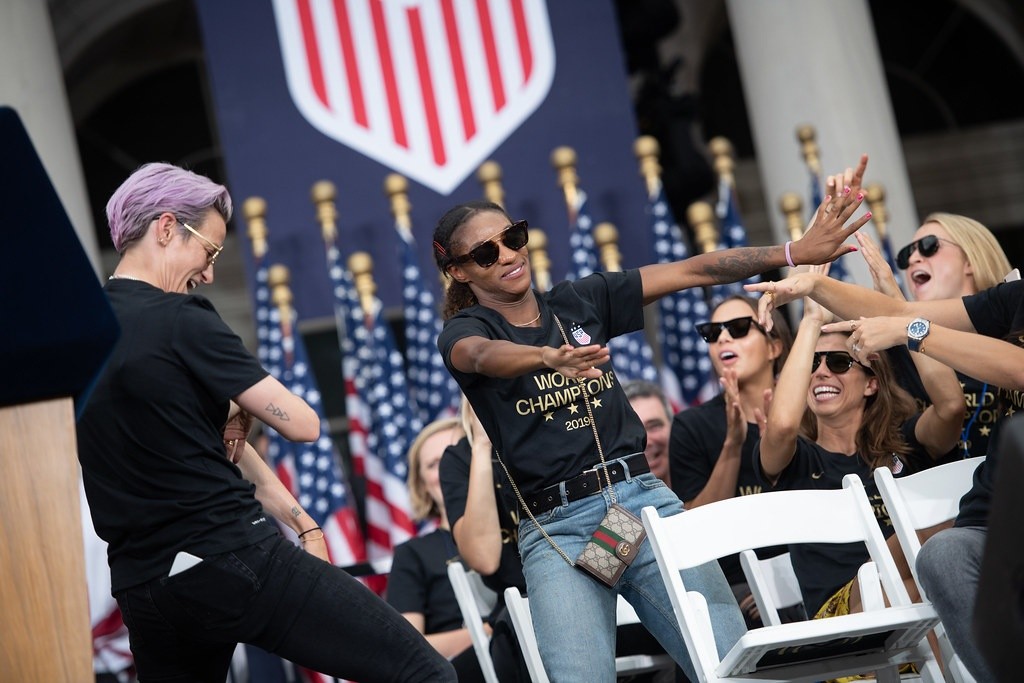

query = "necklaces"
[507,313,541,328]
[109,275,159,287]
[960,383,991,460]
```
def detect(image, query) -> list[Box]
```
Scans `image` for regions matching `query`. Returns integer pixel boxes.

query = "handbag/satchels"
[576,502,647,587]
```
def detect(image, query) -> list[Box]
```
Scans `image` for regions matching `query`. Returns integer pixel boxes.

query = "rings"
[852,331,855,340]
[769,281,776,291]
[227,440,235,446]
[853,345,861,352]
[850,320,856,330]
[765,292,774,301]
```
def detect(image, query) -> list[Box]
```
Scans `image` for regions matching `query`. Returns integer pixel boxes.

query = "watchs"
[905,317,931,353]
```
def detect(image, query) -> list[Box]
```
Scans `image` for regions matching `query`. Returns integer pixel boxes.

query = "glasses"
[694,316,767,343]
[175,219,223,264]
[447,219,529,268]
[894,234,963,270]
[811,350,876,376]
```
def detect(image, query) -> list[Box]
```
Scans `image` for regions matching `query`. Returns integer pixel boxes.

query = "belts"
[519,452,651,518]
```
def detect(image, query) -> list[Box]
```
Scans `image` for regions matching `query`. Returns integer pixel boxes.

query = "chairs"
[641,474,949,683]
[504,586,677,683]
[740,550,923,683]
[448,561,501,683]
[874,455,988,683]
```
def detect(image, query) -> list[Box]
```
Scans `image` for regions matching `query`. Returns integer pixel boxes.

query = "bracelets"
[784,241,797,268]
[825,210,839,219]
[298,526,324,543]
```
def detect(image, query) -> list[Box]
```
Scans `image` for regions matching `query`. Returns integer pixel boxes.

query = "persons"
[386,384,529,683]
[74,161,458,683]
[621,152,1024,682]
[431,187,873,682]
